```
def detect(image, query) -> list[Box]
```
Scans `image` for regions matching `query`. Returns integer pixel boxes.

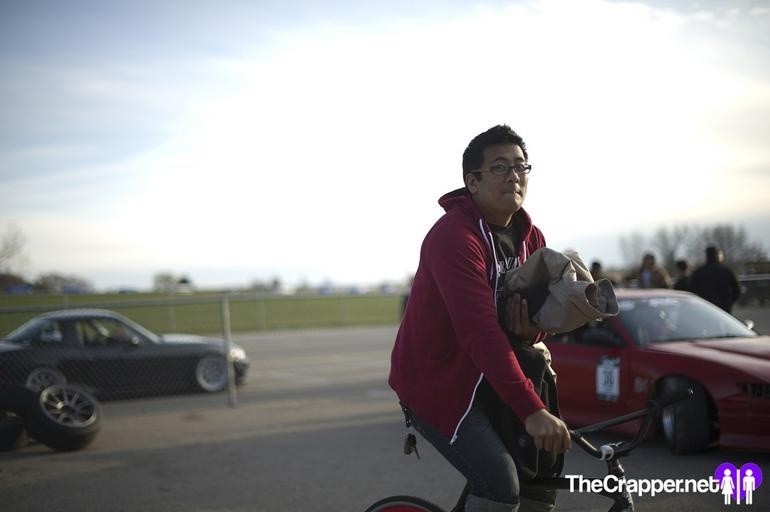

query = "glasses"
[470,162,532,177]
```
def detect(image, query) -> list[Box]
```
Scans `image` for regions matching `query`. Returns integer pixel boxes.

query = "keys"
[399,404,420,460]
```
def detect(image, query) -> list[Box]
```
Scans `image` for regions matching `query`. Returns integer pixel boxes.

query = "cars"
[0,308,249,402]
[543,286,769,456]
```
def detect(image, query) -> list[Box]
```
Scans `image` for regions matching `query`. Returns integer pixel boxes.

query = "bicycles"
[365,386,693,510]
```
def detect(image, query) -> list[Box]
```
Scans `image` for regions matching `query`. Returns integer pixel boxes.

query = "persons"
[624,247,740,313]
[590,263,616,285]
[388,124,572,512]
